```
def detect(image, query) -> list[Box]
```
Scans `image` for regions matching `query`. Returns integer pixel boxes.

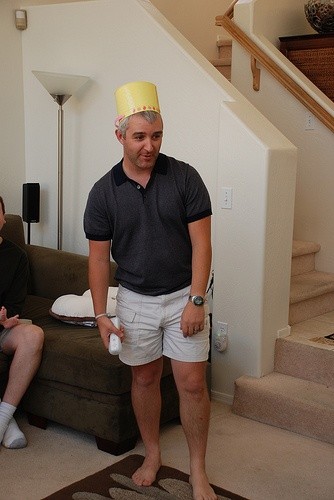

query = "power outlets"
[217,322,229,334]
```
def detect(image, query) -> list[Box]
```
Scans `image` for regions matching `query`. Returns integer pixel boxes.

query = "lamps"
[32,67,90,255]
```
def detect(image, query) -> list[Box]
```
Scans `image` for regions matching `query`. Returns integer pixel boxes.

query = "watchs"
[188,293,207,308]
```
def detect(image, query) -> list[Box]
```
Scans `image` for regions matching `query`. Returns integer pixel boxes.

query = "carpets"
[41,454,250,500]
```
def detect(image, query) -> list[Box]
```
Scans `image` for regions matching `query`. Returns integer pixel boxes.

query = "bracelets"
[95,313,108,320]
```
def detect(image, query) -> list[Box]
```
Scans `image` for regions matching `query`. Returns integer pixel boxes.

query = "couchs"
[1,213,213,456]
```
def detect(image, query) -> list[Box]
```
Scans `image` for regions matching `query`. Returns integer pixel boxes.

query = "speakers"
[22,183,40,224]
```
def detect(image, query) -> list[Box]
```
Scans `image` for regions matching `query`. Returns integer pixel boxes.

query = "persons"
[75,81,220,500]
[0,195,45,451]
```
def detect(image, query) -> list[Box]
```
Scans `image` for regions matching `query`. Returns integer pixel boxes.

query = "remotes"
[107,317,122,355]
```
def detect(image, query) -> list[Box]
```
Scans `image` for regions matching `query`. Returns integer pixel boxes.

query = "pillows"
[49,286,117,329]
